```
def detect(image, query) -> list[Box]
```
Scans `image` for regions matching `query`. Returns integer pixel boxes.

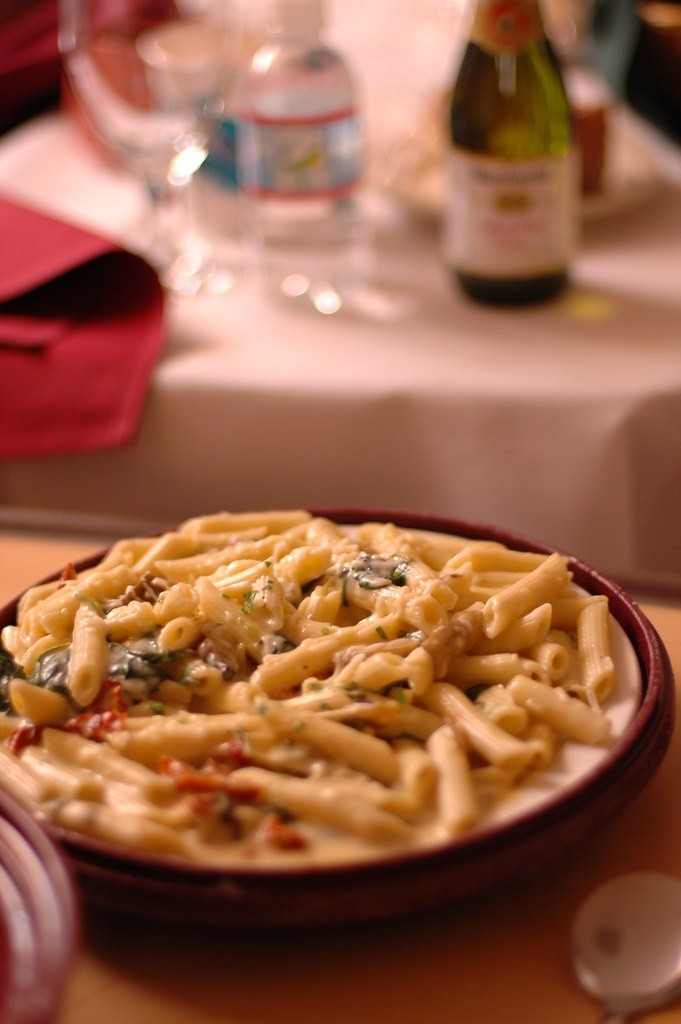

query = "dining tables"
[2,52,681,1024]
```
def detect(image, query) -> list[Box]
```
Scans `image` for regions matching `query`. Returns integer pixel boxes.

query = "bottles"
[441,1,580,309]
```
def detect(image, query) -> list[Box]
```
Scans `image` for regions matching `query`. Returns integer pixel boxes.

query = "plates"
[0,506,675,930]
[378,127,661,226]
[0,777,82,1024]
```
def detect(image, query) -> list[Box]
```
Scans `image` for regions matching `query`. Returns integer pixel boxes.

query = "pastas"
[0,507,615,863]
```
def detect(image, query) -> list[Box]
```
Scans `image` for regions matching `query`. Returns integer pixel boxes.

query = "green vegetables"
[0,551,422,708]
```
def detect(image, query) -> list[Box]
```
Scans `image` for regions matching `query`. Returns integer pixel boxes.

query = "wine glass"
[55,0,246,304]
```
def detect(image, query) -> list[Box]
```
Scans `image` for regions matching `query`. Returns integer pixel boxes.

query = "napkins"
[0,193,163,448]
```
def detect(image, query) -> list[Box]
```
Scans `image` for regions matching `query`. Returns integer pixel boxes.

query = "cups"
[237,0,367,320]
